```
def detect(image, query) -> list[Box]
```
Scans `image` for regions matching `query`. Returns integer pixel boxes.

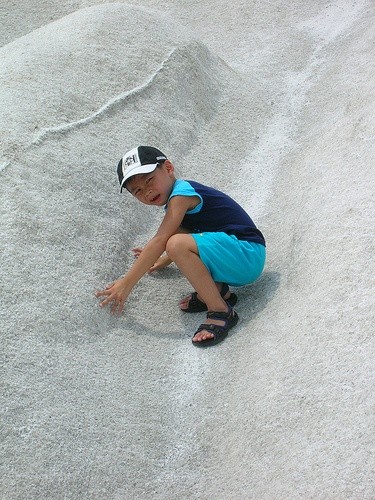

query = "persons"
[93,146,268,349]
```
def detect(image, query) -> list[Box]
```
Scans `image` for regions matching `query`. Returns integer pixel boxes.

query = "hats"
[116,146,168,193]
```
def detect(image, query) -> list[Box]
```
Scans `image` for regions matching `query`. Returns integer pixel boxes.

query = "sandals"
[179,283,237,313]
[193,301,239,348]
